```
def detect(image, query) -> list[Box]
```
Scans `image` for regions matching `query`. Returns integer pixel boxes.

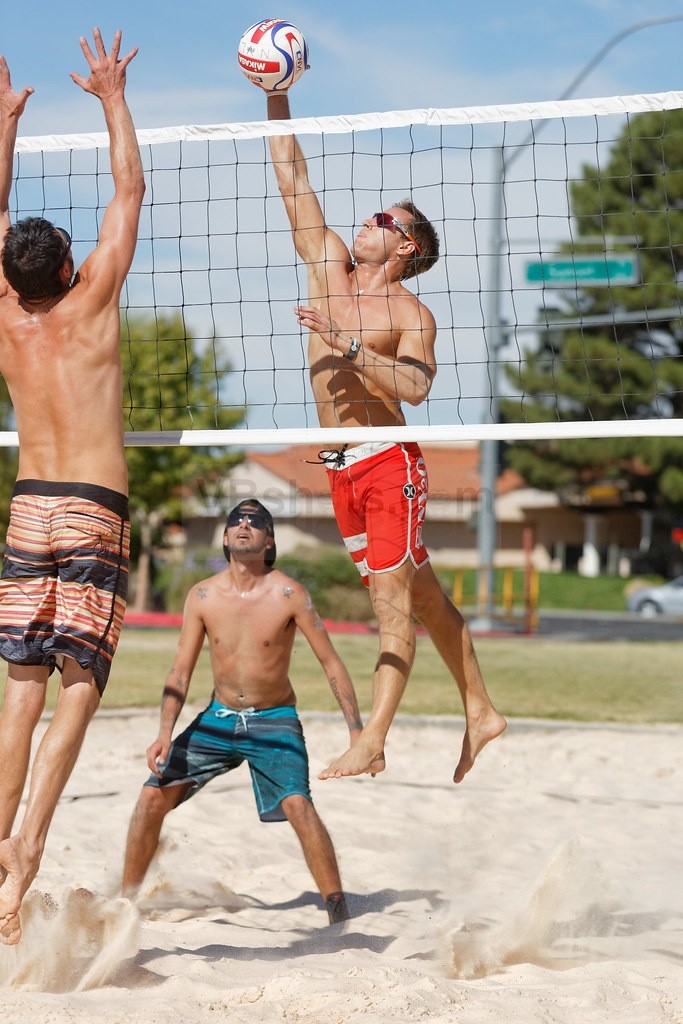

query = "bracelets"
[342,337,361,361]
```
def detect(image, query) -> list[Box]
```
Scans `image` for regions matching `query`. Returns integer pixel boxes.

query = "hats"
[223,500,276,569]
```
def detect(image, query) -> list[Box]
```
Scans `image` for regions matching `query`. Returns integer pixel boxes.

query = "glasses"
[373,213,422,254]
[55,227,72,256]
[227,513,271,533]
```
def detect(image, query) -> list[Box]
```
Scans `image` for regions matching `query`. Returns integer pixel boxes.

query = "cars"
[627,573,683,618]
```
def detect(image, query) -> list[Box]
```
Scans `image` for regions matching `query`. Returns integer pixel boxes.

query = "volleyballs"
[237,17,310,91]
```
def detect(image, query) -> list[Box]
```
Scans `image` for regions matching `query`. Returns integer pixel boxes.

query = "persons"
[123,497,378,928]
[266,90,510,783]
[0,23,147,944]
